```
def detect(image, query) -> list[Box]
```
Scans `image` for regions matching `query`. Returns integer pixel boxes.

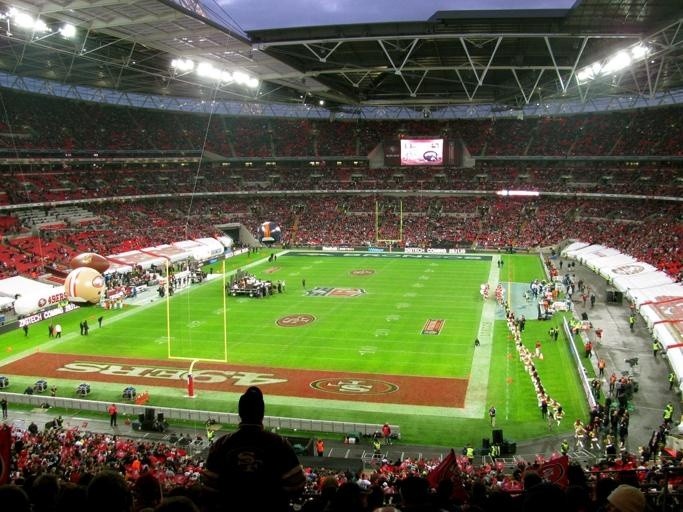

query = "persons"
[0,0,683,512]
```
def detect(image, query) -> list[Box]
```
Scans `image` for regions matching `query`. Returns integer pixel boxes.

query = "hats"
[238,387,263,422]
[607,485,645,512]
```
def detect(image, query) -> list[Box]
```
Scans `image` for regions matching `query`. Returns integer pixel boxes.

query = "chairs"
[0,86,683,512]
